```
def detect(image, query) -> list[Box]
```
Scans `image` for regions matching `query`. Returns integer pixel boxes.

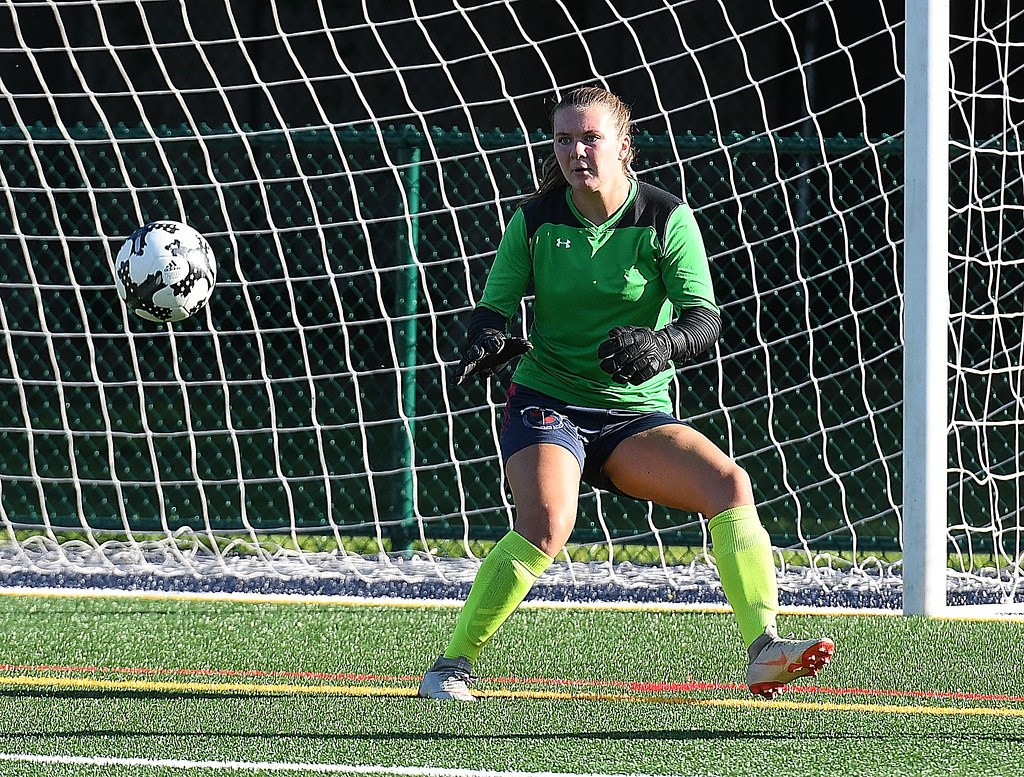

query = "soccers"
[112,219,218,325]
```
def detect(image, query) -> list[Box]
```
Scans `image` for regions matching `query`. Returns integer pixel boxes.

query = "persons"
[418,87,836,703]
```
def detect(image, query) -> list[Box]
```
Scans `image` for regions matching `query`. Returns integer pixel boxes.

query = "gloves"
[450,327,534,388]
[596,325,673,387]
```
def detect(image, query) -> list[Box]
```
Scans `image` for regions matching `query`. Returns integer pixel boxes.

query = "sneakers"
[746,633,835,700]
[416,652,485,703]
